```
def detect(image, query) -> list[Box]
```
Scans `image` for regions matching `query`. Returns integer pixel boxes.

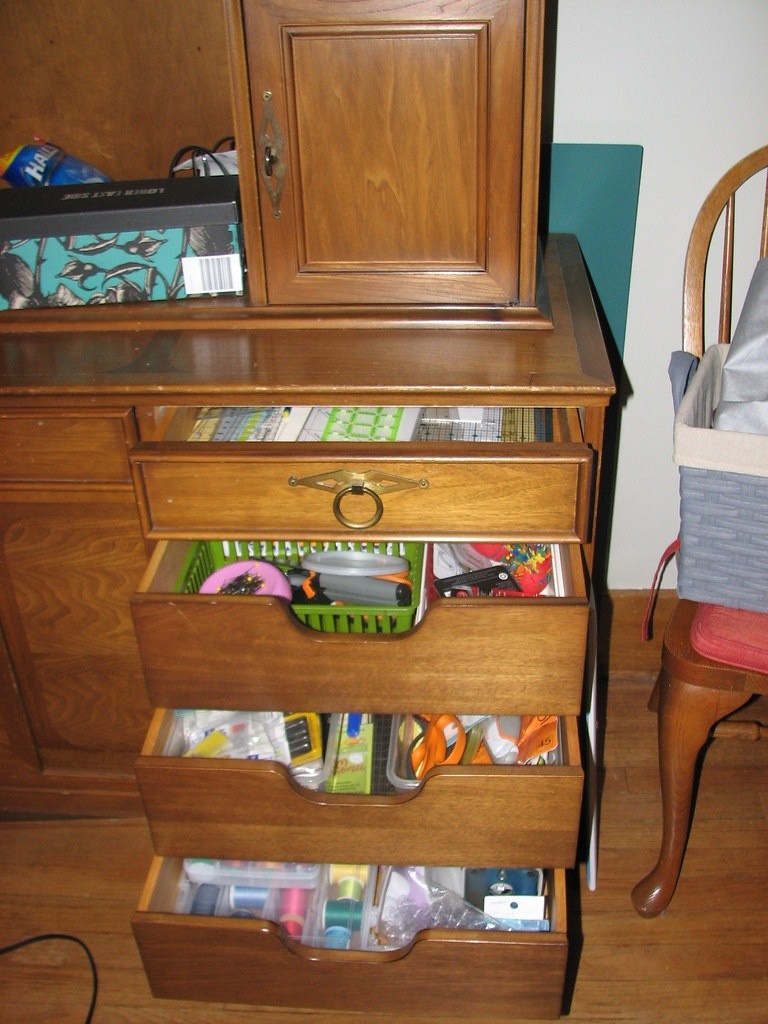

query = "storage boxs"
[162,713,343,793]
[183,858,321,889]
[174,864,380,950]
[385,714,563,794]
[672,342,768,614]
[0,175,248,312]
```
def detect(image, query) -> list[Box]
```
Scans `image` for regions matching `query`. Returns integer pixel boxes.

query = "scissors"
[410,713,467,781]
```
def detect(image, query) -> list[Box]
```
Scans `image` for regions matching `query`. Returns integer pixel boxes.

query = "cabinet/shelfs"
[0,0,554,335]
[0,234,617,1020]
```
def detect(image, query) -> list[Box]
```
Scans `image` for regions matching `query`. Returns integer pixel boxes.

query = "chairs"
[630,145,768,920]
